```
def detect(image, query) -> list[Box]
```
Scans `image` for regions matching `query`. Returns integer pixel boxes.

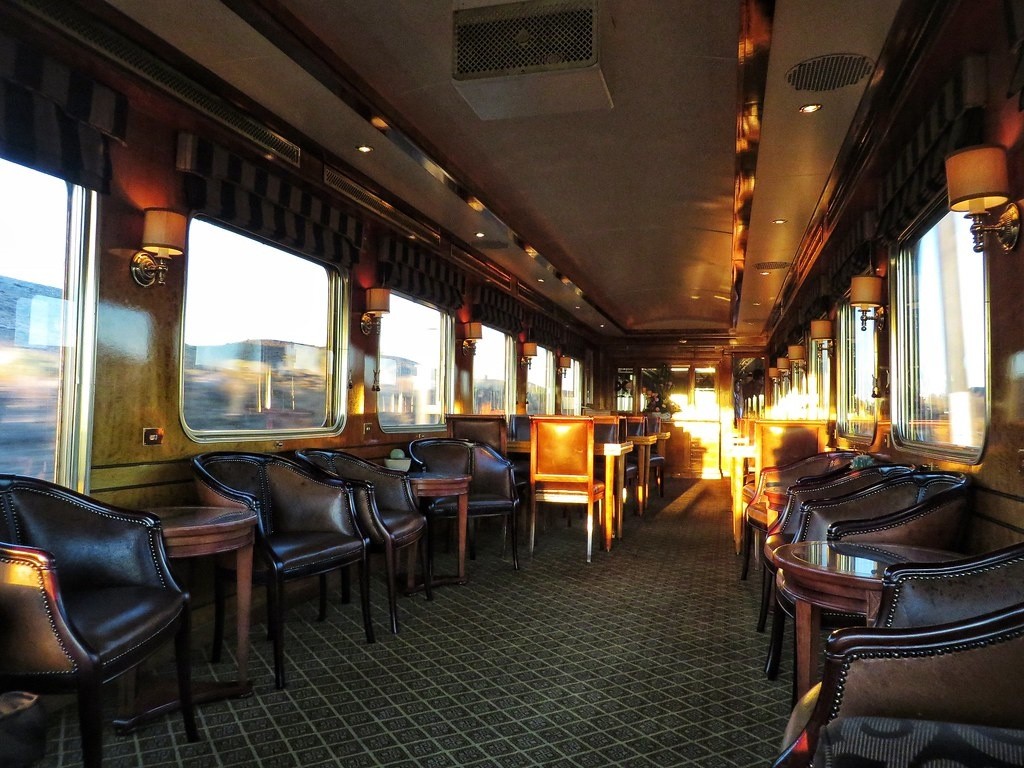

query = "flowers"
[643,386,680,414]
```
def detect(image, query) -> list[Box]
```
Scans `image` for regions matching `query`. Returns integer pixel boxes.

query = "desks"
[112,506,259,731]
[628,436,658,516]
[396,470,473,595]
[506,441,633,553]
[757,486,791,633]
[646,432,672,511]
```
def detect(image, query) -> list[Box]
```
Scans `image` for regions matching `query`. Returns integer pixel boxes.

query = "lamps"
[558,356,571,379]
[787,343,807,377]
[462,322,482,357]
[810,319,834,359]
[520,342,538,370]
[769,366,782,387]
[945,144,1022,252]
[850,274,885,332]
[362,287,391,337]
[777,357,792,383]
[130,208,188,287]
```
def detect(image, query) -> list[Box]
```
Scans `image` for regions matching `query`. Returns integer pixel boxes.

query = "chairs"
[773,542,1024,768]
[729,417,1024,767]
[445,411,664,563]
[0,436,520,768]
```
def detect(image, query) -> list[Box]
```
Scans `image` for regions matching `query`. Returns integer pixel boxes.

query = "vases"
[652,412,671,419]
